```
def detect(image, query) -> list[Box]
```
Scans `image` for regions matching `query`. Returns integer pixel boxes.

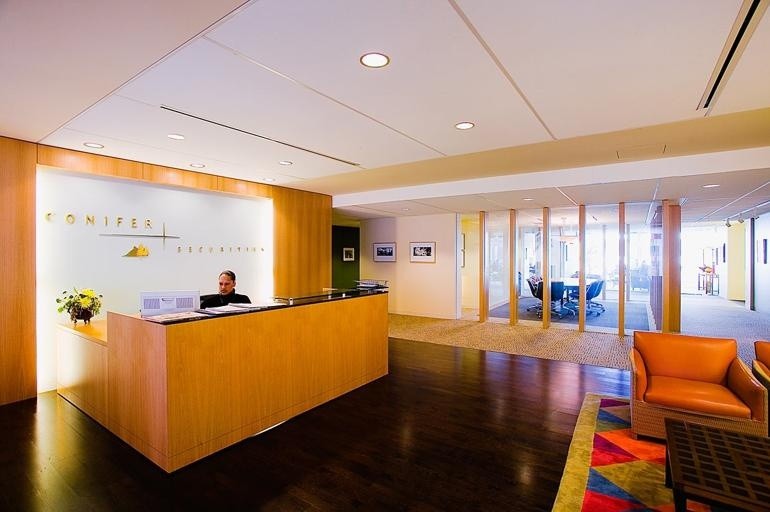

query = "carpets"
[477,295,650,331]
[551,392,709,512]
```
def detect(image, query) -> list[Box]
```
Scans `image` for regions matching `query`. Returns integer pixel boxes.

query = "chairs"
[628,331,768,441]
[751,341,770,388]
[527,273,604,320]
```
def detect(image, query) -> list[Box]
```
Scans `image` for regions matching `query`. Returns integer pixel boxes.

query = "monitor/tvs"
[140,290,200,316]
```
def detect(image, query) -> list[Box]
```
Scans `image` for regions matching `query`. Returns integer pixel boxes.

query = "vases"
[72,306,93,325]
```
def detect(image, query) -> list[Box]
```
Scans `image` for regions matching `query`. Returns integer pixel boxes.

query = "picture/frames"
[343,247,354,261]
[373,243,397,262]
[409,241,436,262]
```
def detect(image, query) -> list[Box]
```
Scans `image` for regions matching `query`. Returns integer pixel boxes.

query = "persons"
[199,270,251,308]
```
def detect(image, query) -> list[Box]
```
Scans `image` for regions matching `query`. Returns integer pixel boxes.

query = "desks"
[698,272,720,296]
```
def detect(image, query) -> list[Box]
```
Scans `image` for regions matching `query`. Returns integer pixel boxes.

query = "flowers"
[54,286,102,322]
